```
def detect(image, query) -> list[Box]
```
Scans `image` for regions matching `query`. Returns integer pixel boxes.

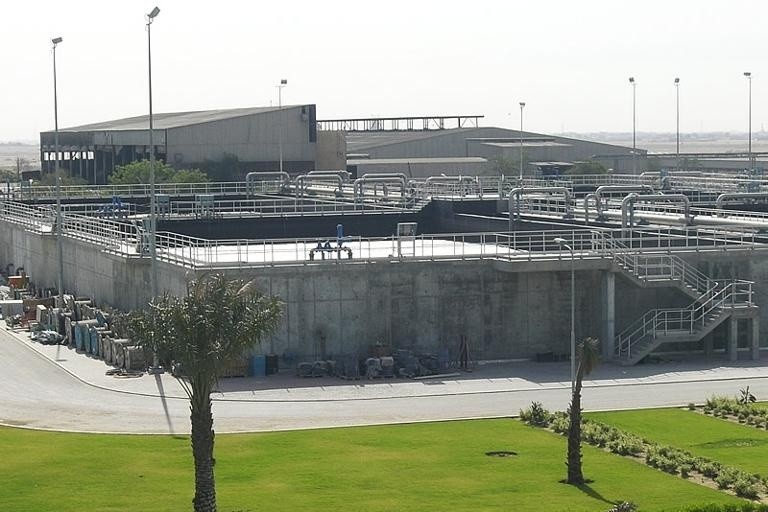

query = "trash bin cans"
[253,355,266,378]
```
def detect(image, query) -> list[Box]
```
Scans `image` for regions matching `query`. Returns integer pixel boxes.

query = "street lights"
[674,77,682,164]
[628,76,638,175]
[277,77,288,183]
[50,37,64,309]
[742,71,753,175]
[550,232,576,399]
[144,5,162,375]
[518,102,529,186]
